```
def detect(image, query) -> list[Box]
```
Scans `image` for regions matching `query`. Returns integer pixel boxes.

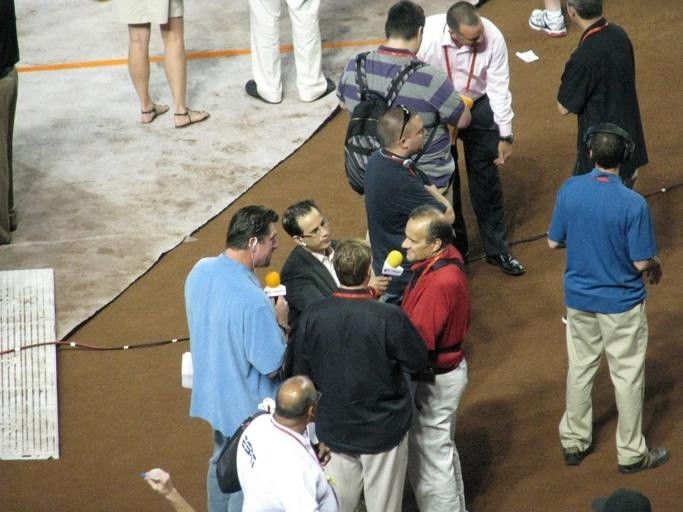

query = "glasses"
[315,390,323,406]
[296,218,331,238]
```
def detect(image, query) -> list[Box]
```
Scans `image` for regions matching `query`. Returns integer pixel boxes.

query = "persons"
[400,217,469,512]
[143,469,196,511]
[364,106,456,280]
[0,0,23,245]
[241,0,337,108]
[547,126,671,473]
[108,1,209,130]
[184,203,293,512]
[556,3,649,193]
[269,198,394,383]
[590,488,652,512]
[298,239,430,512]
[237,375,339,512]
[415,1,526,276]
[528,0,567,37]
[339,0,471,197]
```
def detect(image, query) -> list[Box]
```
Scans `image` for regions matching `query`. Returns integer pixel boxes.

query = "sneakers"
[529,9,567,37]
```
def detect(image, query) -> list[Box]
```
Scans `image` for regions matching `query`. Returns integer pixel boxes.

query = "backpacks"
[343,50,430,196]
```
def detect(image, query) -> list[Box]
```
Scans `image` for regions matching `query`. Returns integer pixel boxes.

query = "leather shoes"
[314,76,337,100]
[246,79,269,102]
[485,251,525,276]
[464,253,474,278]
[618,447,671,474]
[565,440,595,464]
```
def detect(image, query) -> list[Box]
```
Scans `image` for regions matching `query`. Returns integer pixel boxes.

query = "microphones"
[382,249,404,277]
[264,271,287,303]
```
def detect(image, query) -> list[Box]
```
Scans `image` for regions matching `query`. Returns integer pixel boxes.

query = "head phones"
[582,124,636,163]
[382,148,414,169]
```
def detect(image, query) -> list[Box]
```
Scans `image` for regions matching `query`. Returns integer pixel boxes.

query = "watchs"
[276,319,291,335]
[498,135,515,143]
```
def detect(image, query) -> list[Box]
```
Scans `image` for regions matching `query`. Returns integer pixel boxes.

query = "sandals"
[141,103,169,124]
[0,206,18,244]
[176,106,210,129]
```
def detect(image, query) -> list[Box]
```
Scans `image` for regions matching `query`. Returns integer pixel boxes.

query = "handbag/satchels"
[216,411,270,493]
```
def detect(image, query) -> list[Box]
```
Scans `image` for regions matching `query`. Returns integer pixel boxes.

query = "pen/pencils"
[137,471,160,482]
